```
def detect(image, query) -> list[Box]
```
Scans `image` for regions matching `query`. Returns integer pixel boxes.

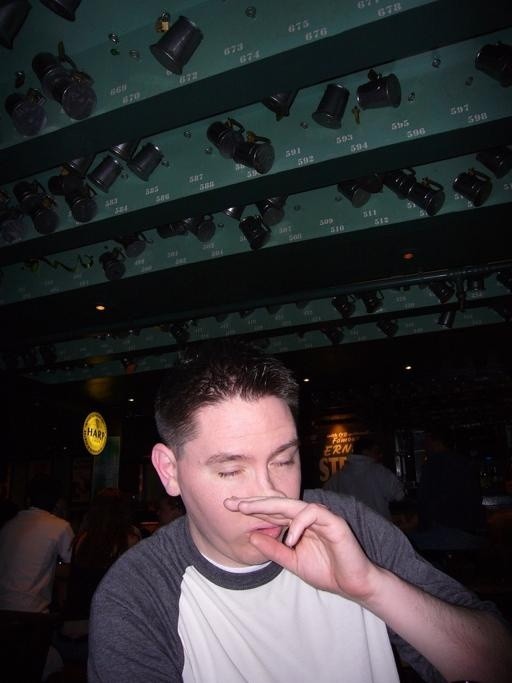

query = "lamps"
[436,309,457,330]
[204,116,245,158]
[108,137,142,161]
[360,290,385,313]
[262,86,301,121]
[29,202,58,234]
[321,327,346,344]
[221,205,245,222]
[147,13,205,77]
[451,169,494,208]
[382,167,417,199]
[231,132,277,176]
[355,73,406,110]
[473,37,510,91]
[88,157,124,193]
[33,41,100,121]
[376,320,400,338]
[239,216,270,249]
[46,174,84,197]
[3,71,46,139]
[476,147,511,179]
[64,187,97,223]
[407,179,443,217]
[330,295,357,317]
[168,322,190,343]
[12,179,46,209]
[155,212,219,242]
[255,197,290,224]
[310,81,350,132]
[96,249,125,282]
[336,170,381,208]
[62,154,95,177]
[428,277,455,302]
[119,233,150,259]
[128,143,164,183]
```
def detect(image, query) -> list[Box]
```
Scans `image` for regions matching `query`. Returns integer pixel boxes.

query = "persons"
[49,486,142,682]
[87,345,512,683]
[0,468,82,683]
[320,421,481,590]
